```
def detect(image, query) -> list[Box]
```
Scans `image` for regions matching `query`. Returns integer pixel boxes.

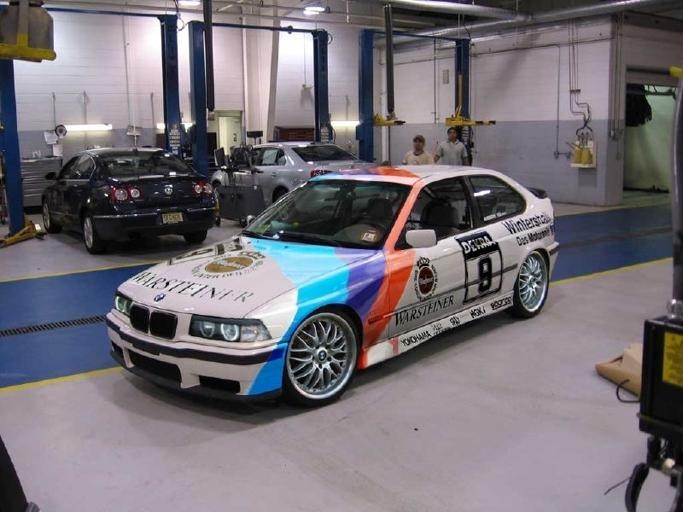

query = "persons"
[401,134,435,168]
[431,126,471,168]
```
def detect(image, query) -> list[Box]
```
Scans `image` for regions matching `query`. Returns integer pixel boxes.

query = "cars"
[105,162,560,407]
[39,146,223,254]
[211,140,378,227]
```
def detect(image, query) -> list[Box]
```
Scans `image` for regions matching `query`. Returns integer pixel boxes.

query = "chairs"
[342,195,458,247]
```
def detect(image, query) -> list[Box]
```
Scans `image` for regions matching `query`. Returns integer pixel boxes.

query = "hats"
[413,135,425,142]
